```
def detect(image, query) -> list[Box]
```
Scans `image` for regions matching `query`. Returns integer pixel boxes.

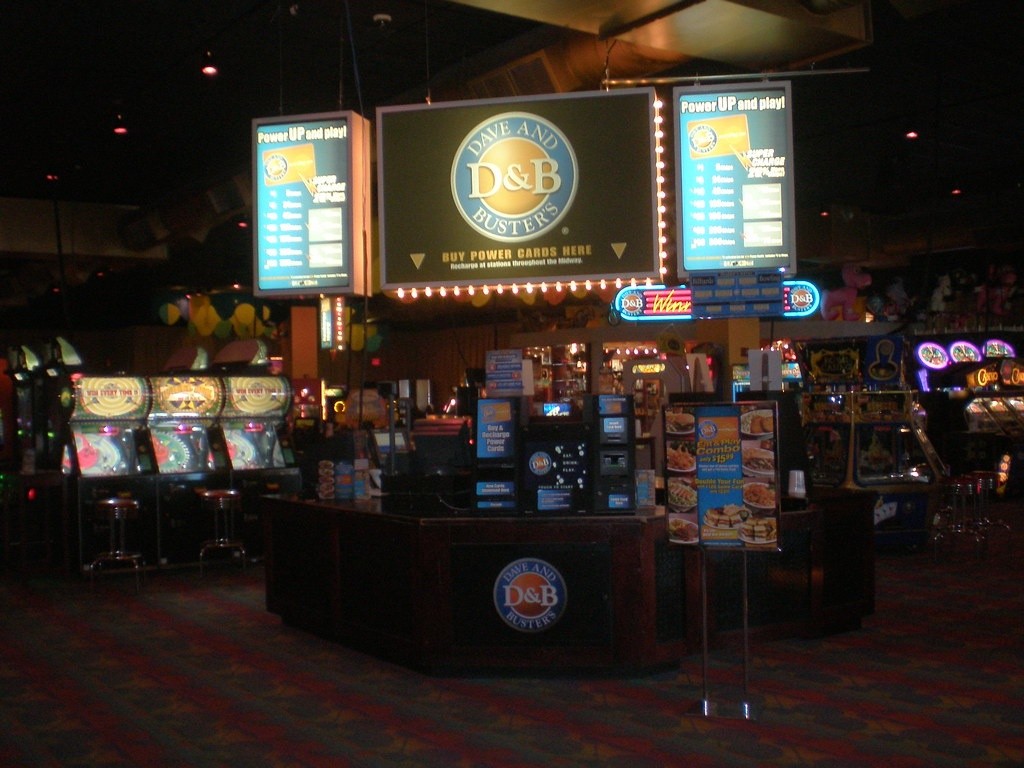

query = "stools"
[89,499,148,595]
[966,471,1012,562]
[194,486,247,586]
[933,475,985,569]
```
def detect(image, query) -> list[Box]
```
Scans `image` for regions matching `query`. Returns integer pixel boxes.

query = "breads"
[751,415,774,433]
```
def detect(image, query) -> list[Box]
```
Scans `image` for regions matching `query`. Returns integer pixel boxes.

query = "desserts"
[703,508,736,528]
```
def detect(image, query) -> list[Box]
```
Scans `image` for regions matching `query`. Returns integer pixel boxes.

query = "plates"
[737,518,777,544]
[669,502,697,513]
[704,521,740,530]
[740,409,773,437]
[743,482,775,509]
[741,447,774,483]
[667,460,696,473]
[669,517,699,544]
[665,426,695,435]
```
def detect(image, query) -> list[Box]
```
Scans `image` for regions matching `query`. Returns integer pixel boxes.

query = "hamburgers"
[673,413,695,431]
